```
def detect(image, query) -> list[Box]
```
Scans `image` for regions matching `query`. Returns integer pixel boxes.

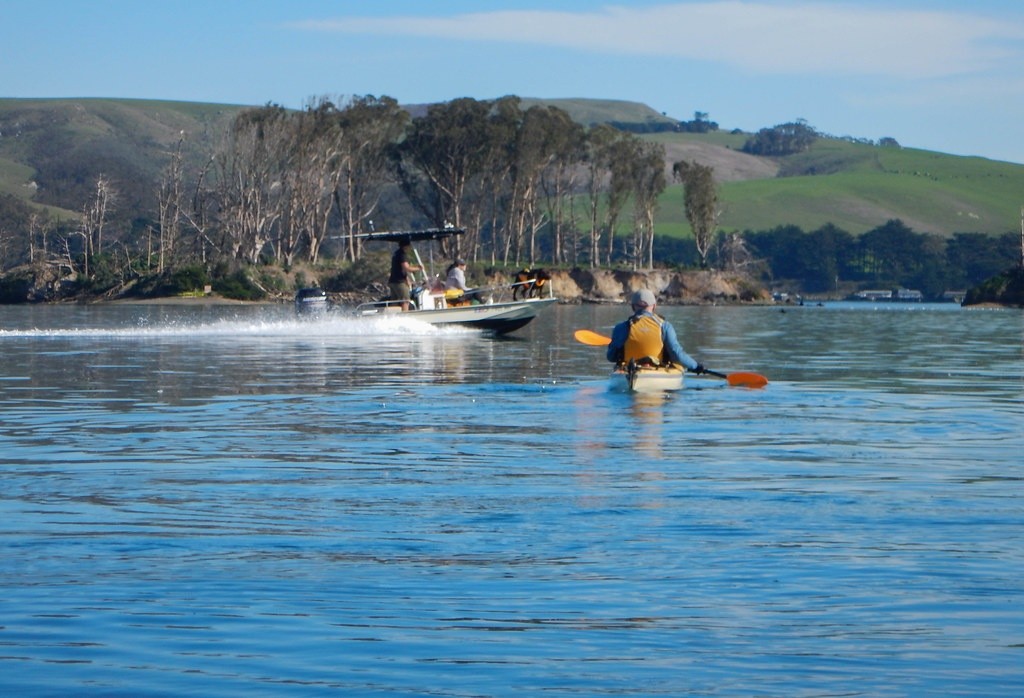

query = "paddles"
[574,329,769,389]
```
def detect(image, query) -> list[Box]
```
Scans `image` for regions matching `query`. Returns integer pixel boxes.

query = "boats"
[611,357,685,393]
[355,226,558,335]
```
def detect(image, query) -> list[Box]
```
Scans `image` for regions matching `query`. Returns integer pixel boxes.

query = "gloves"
[689,363,702,373]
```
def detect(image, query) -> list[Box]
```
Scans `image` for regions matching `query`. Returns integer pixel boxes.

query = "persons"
[444,258,486,304]
[605,289,704,376]
[388,240,422,309]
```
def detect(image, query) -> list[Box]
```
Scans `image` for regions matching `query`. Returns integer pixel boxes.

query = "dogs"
[510,267,551,300]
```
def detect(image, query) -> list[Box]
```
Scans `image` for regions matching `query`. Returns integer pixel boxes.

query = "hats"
[399,239,410,246]
[454,259,466,266]
[631,288,656,307]
